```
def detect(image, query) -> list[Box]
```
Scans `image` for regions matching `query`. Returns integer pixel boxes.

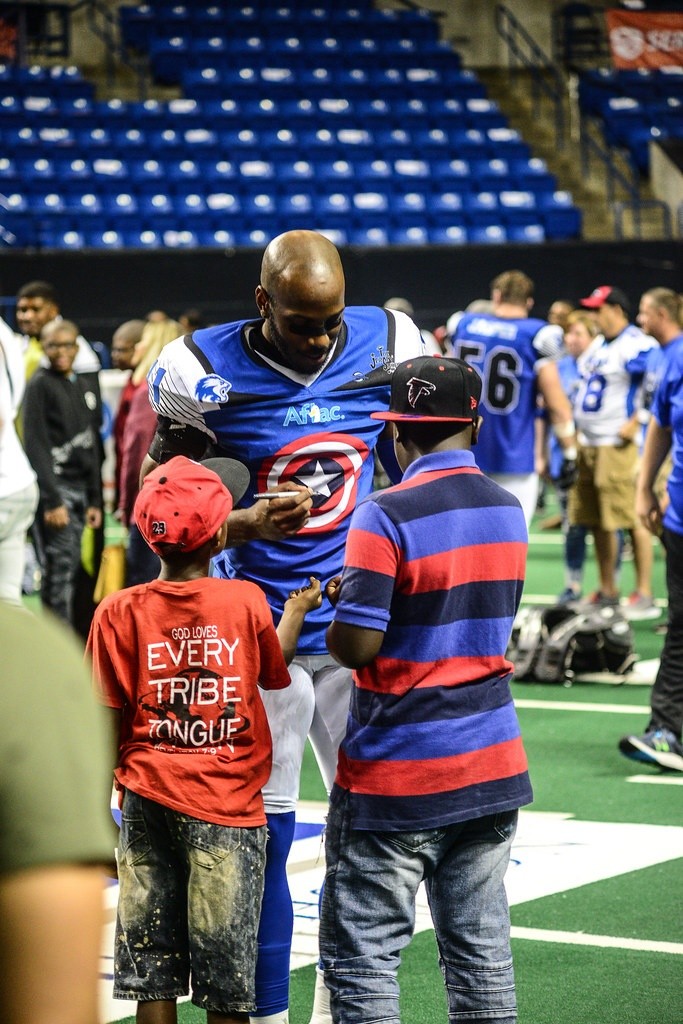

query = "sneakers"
[618,729,683,769]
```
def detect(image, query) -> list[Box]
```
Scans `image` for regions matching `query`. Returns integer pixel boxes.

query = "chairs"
[577,0,683,213]
[1,0,583,247]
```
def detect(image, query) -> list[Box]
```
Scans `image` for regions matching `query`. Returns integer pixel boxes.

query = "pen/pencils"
[253,492,322,499]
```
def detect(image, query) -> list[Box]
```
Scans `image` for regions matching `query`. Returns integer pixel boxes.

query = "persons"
[1,281,207,633]
[137,231,426,1023]
[380,267,683,772]
[317,354,528,1024]
[84,454,322,1023]
[1,599,117,1024]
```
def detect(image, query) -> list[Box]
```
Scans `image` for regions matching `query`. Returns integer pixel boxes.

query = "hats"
[133,455,250,557]
[370,354,482,421]
[580,285,630,312]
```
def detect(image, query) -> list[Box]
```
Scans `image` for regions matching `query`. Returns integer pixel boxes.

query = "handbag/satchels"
[506,608,634,683]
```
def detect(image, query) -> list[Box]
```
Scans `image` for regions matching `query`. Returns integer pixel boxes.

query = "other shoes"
[589,592,619,607]
[623,592,662,621]
[557,588,582,606]
